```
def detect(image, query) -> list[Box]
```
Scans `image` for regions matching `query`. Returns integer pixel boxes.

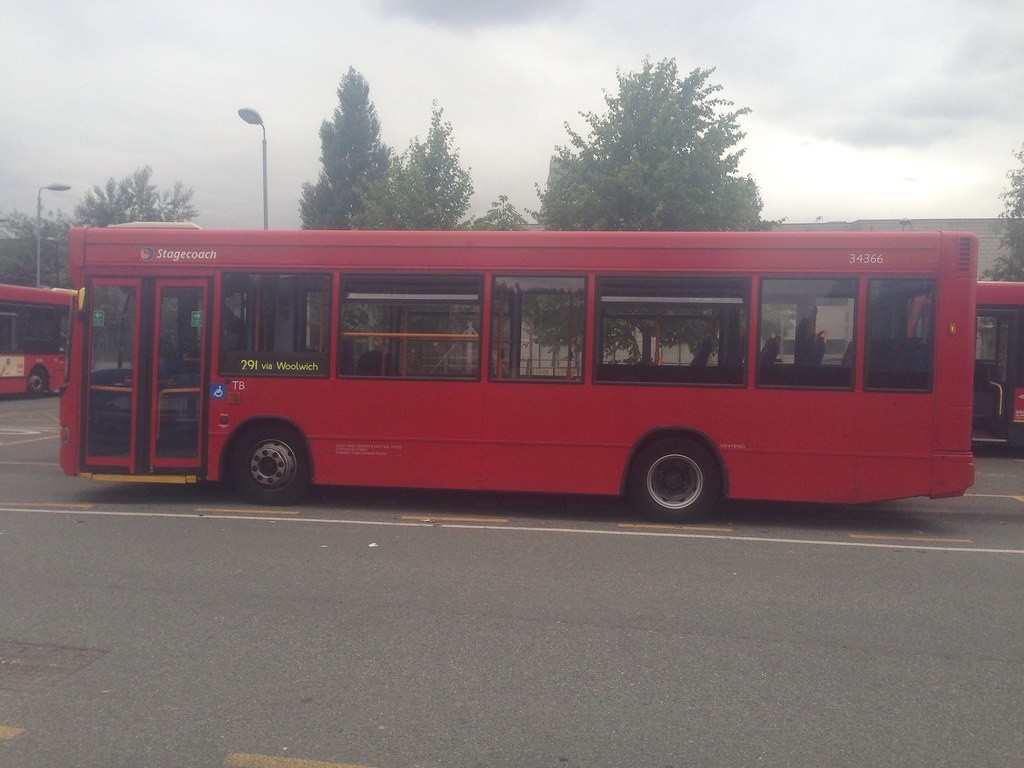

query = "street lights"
[237,107,269,231]
[35,183,71,288]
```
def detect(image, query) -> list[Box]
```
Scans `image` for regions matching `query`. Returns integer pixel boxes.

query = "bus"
[0,282,73,397]
[59,220,1023,525]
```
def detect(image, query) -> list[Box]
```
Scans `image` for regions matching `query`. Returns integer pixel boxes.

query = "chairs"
[357,350,393,376]
[691,337,712,368]
[759,336,781,366]
[798,330,829,366]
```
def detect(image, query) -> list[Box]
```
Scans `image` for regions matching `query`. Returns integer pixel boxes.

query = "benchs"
[841,334,928,368]
